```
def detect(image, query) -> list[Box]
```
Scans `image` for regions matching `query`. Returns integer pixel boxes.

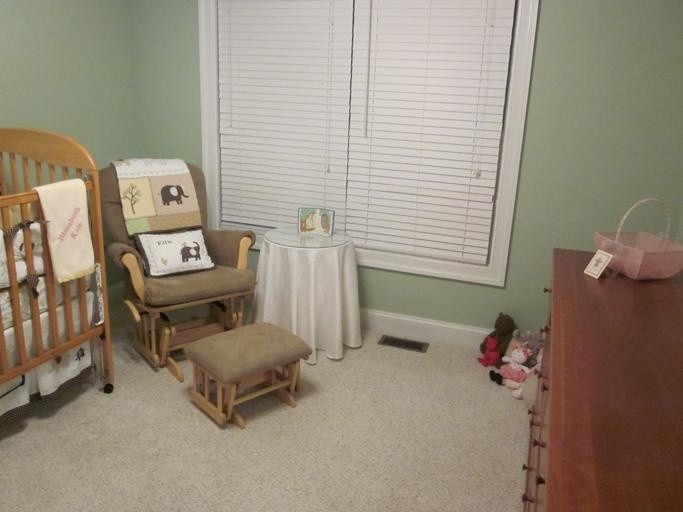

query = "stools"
[182,321,314,428]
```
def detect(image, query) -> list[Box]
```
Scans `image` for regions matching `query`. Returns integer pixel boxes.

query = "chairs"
[98,158,258,383]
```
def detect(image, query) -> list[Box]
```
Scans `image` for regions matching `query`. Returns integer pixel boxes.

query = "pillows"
[134,225,216,277]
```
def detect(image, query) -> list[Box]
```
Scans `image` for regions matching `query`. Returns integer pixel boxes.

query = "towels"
[111,158,203,238]
[32,178,95,285]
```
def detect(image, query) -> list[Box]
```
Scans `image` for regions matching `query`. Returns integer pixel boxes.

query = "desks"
[263,227,353,357]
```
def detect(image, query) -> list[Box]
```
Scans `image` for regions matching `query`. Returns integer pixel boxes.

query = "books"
[298,207,337,237]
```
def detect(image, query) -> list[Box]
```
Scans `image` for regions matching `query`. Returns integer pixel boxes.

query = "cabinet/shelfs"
[520,246,682,512]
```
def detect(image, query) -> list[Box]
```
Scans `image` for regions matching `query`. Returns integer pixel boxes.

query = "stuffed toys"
[0,219,46,291]
[475,309,545,411]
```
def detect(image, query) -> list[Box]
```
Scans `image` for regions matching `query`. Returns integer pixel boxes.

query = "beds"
[0,127,114,395]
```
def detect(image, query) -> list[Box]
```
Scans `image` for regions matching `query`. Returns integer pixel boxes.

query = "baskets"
[595,198,683,279]
